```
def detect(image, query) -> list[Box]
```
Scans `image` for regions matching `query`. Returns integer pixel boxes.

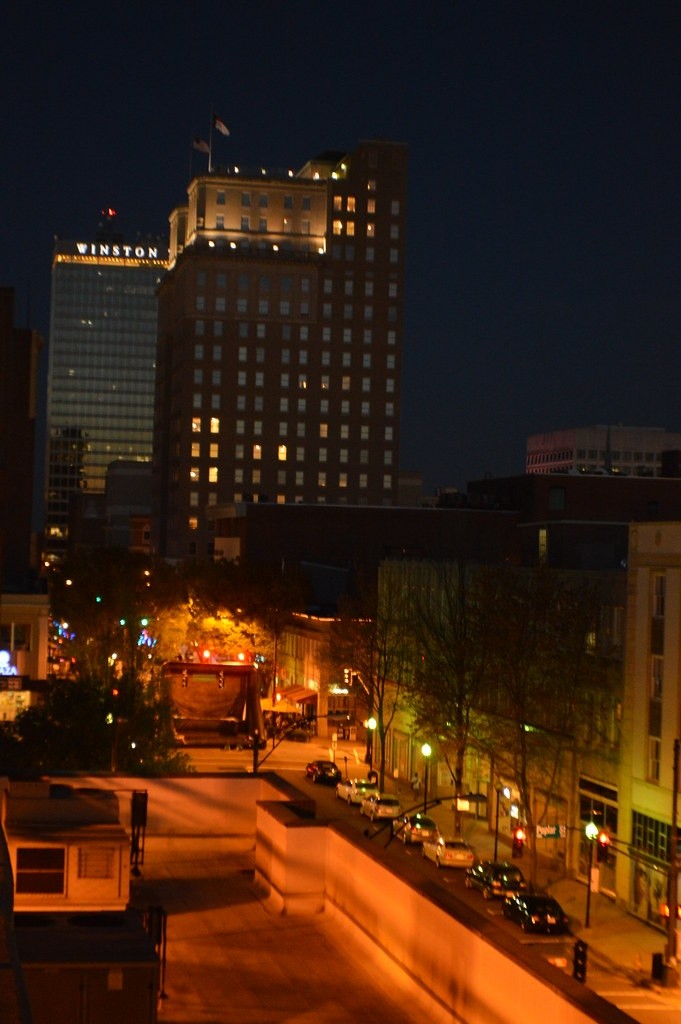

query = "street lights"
[421,743,432,817]
[367,717,378,771]
[585,820,599,927]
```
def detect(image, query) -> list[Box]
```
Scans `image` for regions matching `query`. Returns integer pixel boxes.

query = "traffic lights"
[343,668,352,687]
[513,825,526,850]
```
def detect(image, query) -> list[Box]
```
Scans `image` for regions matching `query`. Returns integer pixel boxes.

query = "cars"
[392,814,439,847]
[335,777,380,806]
[360,791,402,823]
[503,891,567,934]
[306,760,342,785]
[466,858,524,902]
[421,832,474,868]
